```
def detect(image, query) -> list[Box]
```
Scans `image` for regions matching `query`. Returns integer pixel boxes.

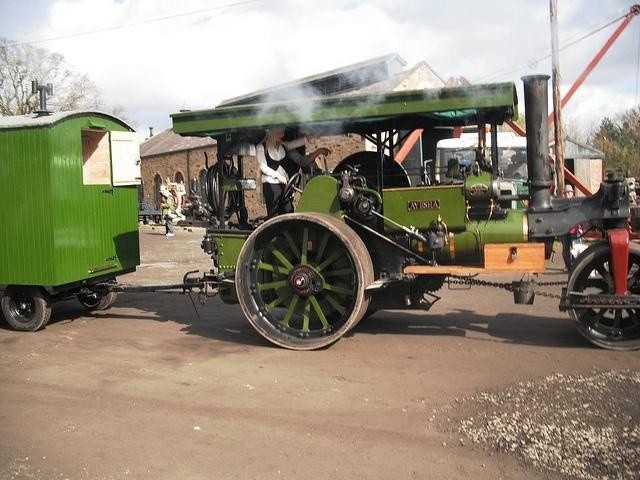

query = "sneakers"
[165,232,175,237]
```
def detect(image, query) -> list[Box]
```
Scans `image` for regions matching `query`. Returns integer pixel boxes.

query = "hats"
[563,184,575,193]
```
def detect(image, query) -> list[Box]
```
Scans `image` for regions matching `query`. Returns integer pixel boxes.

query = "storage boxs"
[484,242,546,273]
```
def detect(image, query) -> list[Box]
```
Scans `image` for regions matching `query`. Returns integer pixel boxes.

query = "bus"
[421,132,528,209]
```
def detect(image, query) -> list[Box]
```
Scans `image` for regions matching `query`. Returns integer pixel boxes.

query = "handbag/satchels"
[167,211,179,219]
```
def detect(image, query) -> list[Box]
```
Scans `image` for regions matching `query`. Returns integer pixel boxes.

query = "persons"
[256,124,331,216]
[160,189,175,236]
[560,184,582,274]
